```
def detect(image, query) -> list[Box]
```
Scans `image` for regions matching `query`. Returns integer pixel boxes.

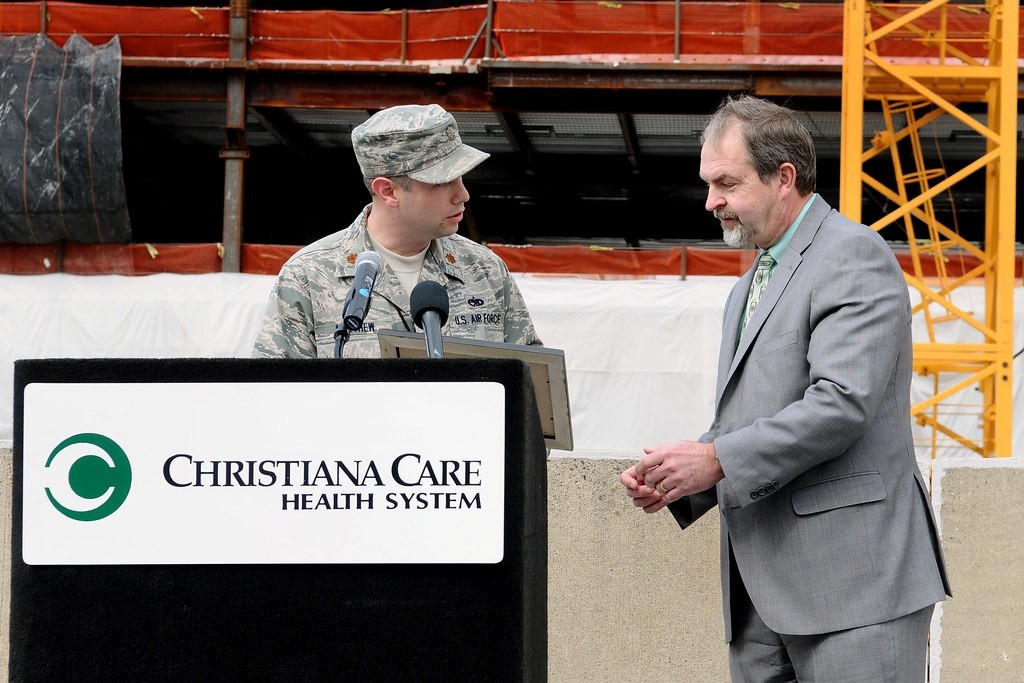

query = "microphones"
[344,252,382,331]
[410,281,450,358]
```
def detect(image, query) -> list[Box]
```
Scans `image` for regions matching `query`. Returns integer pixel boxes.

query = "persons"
[248,102,545,359]
[617,91,953,682]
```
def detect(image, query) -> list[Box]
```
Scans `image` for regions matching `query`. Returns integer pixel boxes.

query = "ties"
[740,252,775,340]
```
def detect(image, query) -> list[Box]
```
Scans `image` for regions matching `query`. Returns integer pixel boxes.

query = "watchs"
[715,452,720,463]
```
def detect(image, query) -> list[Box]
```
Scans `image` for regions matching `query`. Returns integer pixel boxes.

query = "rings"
[660,481,669,492]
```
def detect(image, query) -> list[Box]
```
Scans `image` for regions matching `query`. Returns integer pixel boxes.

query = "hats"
[351,103,491,184]
